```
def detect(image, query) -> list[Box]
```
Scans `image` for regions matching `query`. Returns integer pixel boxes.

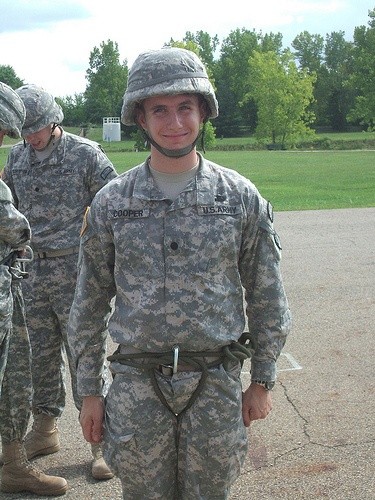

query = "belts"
[119,344,220,366]
[33,245,81,260]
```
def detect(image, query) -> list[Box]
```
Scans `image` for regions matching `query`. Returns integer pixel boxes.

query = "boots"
[89,440,114,480]
[0,442,69,495]
[21,413,58,460]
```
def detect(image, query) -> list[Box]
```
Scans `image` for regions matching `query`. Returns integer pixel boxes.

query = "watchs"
[253,380,275,391]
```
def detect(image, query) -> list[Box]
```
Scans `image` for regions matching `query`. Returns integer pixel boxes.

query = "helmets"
[15,84,64,136]
[120,48,219,127]
[0,82,26,139]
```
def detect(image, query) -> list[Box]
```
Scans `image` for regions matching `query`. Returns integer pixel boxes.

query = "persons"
[0,85,120,479]
[67,47,292,500]
[0,81,68,496]
